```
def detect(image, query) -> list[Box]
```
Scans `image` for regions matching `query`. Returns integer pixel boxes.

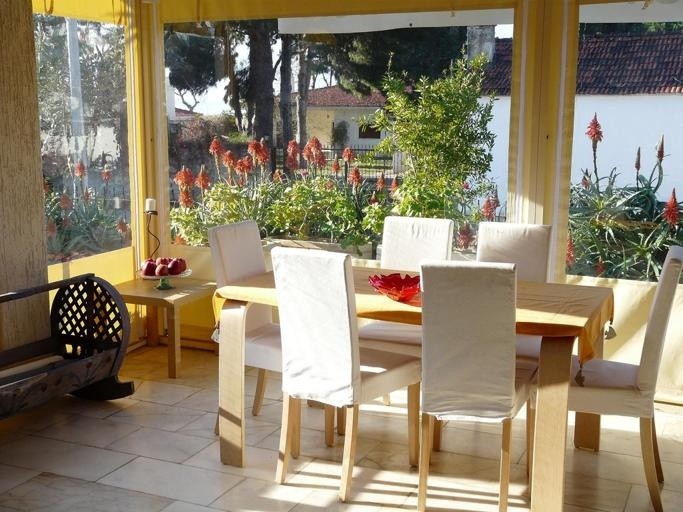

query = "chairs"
[528,244,681,511]
[355,216,455,406]
[208,220,348,446]
[417,263,531,512]
[269,246,423,505]
[476,221,551,356]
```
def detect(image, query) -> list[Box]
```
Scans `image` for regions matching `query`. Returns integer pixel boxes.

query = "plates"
[134,265,192,292]
[368,272,420,303]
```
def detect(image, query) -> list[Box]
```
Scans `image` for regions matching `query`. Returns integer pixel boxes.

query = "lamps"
[493,22,514,44]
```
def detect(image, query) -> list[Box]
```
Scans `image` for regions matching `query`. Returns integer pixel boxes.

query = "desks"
[110,278,218,379]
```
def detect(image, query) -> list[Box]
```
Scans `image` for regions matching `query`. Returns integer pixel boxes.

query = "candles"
[144,197,156,213]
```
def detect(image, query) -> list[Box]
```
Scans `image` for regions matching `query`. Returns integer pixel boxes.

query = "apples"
[144,261,157,276]
[156,257,169,266]
[154,264,168,276]
[175,258,186,272]
[168,261,181,275]
